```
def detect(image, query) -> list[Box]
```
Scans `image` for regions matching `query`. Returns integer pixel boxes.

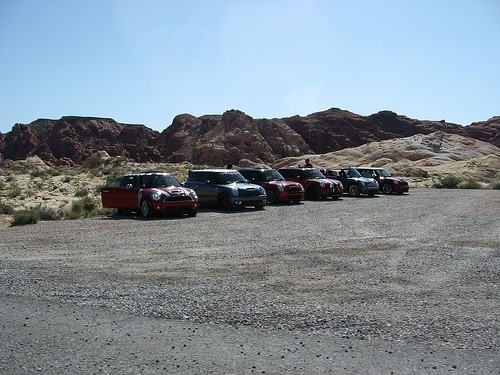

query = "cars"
[101,170,199,217]
[238,169,306,204]
[324,167,379,197]
[185,169,267,210]
[278,168,344,200]
[355,167,410,195]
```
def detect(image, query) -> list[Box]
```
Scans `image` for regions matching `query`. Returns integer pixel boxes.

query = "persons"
[227,163,232,169]
[339,170,352,176]
[298,159,312,168]
[320,167,335,176]
